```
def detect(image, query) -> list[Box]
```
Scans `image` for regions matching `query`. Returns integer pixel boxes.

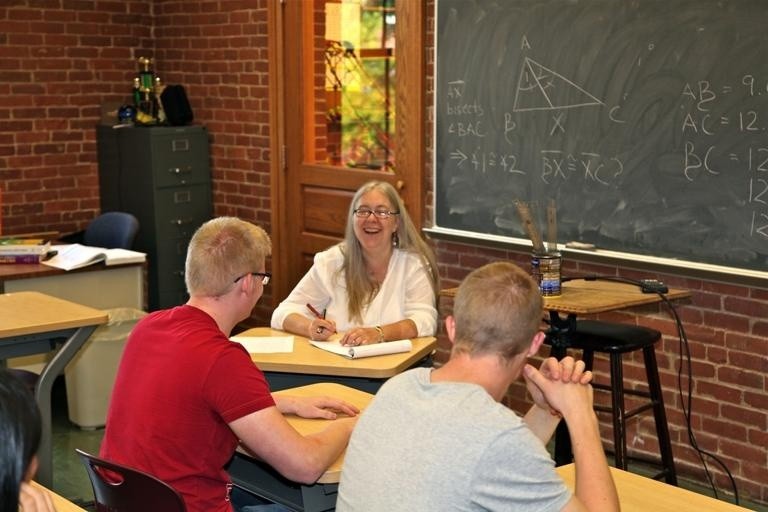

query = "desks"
[437,274,692,470]
[229,326,437,512]
[551,459,754,512]
[0,290,110,488]
[0,245,148,325]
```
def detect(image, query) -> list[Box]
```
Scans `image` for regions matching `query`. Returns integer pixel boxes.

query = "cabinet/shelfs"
[94,123,212,309]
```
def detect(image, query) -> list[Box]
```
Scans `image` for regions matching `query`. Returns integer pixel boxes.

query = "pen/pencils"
[306,303,339,334]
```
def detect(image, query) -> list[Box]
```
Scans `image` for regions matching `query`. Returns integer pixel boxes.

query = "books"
[0,238,52,257]
[39,242,148,271]
[0,250,48,265]
[308,335,414,360]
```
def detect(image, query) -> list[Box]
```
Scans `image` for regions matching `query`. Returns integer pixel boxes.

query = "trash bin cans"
[63,307,151,427]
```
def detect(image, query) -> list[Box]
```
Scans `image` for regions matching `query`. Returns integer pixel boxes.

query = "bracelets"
[374,326,385,343]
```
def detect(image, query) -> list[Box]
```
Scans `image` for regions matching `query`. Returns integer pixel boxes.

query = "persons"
[268,180,440,351]
[0,368,58,510]
[93,216,364,512]
[334,260,622,512]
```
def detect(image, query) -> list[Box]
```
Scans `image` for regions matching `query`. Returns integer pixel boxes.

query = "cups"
[531,253,563,299]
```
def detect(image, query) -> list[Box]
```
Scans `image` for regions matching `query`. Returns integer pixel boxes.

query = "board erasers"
[565,240,596,251]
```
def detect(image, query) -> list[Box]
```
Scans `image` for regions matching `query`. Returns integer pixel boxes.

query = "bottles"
[130,56,166,124]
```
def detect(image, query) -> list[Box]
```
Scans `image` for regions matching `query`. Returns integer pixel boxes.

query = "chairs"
[75,209,139,252]
[75,450,188,512]
[27,478,88,512]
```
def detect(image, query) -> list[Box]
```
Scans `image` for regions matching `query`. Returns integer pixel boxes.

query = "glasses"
[352,208,402,220]
[234,270,272,287]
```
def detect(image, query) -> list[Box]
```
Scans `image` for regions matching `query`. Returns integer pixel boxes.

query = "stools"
[542,319,681,488]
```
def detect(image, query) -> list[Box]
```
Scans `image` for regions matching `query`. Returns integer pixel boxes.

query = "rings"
[316,327,321,334]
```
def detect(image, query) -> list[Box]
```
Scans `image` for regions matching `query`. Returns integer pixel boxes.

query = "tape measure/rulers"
[514,199,558,255]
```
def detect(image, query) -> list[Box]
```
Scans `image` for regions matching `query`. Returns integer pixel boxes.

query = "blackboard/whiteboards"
[434,0,767,282]
